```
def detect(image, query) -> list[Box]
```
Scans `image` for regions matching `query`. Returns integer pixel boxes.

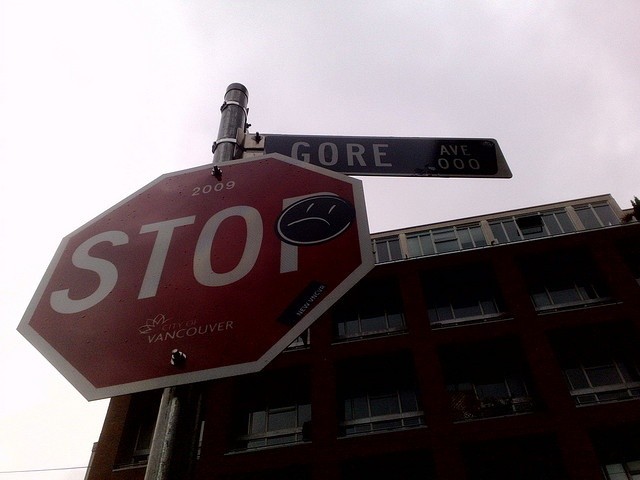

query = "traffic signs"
[264,135,513,178]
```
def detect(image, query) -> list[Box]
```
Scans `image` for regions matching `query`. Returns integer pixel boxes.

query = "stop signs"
[16,152,376,403]
